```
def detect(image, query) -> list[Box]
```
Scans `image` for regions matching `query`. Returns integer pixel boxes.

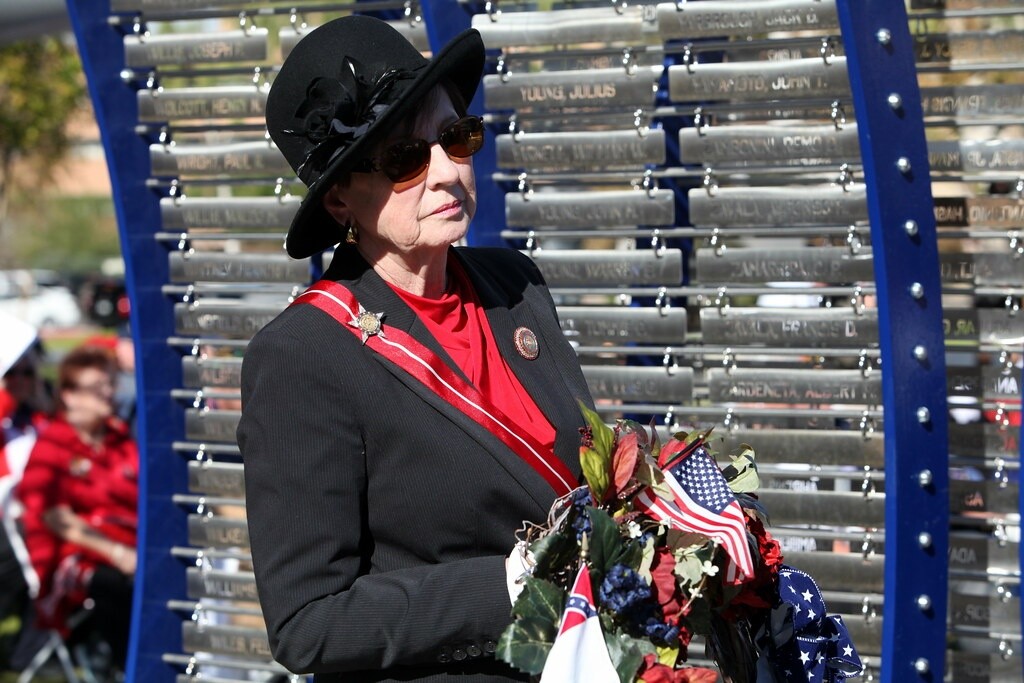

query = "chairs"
[0,495,94,683]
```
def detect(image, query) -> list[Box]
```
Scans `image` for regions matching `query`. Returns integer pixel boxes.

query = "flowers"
[495,395,783,682]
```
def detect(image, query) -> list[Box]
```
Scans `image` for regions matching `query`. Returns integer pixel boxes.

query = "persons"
[0,321,141,683]
[236,14,604,683]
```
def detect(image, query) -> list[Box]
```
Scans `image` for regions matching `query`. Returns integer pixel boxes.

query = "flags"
[540,565,622,683]
[633,441,754,577]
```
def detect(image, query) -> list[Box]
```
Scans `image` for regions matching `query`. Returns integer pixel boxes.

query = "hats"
[265,15,485,260]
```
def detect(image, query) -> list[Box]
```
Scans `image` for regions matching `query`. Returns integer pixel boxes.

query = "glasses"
[349,115,484,184]
